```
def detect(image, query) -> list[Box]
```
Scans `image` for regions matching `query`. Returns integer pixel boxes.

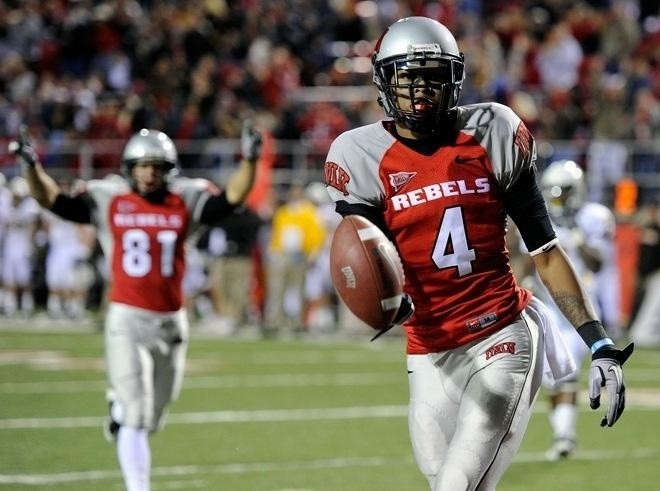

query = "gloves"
[13,124,37,170]
[588,342,634,427]
[240,120,262,161]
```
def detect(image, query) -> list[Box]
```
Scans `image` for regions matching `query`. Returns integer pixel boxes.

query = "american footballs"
[329,215,406,330]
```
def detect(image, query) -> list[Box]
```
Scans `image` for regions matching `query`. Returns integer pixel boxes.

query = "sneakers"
[104,389,120,442]
[551,435,574,459]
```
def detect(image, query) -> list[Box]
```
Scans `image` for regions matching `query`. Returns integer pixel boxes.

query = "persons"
[1,1,660,349]
[508,160,622,464]
[321,13,627,491]
[6,118,267,491]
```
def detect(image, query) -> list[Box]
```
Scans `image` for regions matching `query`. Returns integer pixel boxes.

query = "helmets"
[119,129,179,165]
[543,159,584,217]
[369,15,467,134]
